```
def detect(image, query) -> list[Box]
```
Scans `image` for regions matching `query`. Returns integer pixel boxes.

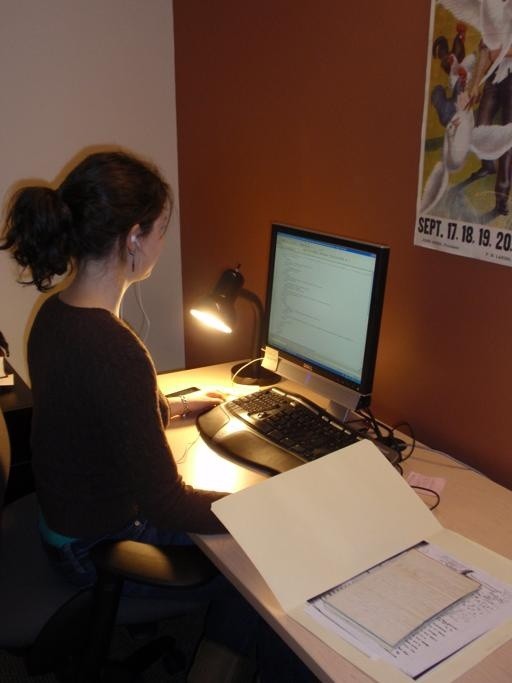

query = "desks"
[156,359,512,682]
[1,356,36,505]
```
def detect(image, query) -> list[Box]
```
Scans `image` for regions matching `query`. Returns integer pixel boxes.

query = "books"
[206,436,512,683]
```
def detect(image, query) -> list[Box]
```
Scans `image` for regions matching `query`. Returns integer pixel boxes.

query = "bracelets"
[179,394,195,415]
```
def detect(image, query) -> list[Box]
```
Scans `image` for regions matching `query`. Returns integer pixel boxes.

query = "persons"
[0,149,317,682]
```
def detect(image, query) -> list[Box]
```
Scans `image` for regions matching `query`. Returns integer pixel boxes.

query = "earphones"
[131,234,142,250]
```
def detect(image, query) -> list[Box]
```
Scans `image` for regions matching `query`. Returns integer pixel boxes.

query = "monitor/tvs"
[257,222,391,424]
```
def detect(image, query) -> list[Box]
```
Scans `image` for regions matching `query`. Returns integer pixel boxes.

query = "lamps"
[188,268,282,387]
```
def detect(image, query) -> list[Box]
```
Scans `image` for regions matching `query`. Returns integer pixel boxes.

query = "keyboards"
[197,385,403,474]
